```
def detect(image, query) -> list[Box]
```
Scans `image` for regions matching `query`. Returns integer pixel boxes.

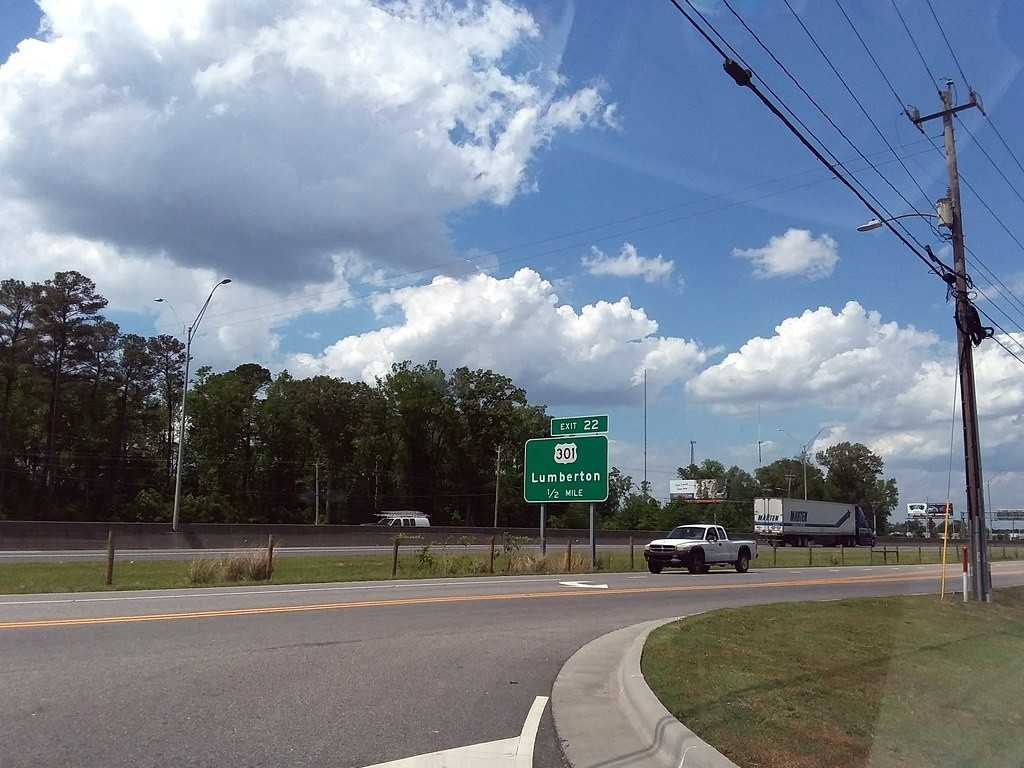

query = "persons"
[708,532,717,541]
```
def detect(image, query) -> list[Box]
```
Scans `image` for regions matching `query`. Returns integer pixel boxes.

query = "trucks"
[755,497,876,548]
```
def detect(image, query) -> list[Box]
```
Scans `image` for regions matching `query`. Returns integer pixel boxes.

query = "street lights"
[856,211,990,601]
[776,425,835,500]
[987,466,1008,542]
[154,278,232,531]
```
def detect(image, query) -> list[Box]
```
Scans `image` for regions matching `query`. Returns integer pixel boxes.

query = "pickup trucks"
[644,524,758,575]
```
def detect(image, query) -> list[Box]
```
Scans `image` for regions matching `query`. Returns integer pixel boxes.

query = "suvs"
[362,516,430,527]
